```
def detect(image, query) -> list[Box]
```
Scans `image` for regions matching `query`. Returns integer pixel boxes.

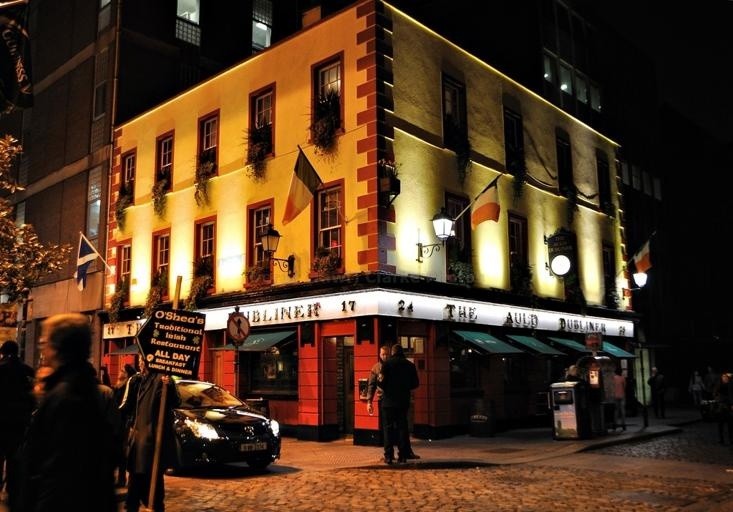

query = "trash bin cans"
[246,399,270,419]
[599,402,616,431]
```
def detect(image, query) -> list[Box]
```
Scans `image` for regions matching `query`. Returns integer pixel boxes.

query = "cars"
[698,393,722,418]
[166,380,281,477]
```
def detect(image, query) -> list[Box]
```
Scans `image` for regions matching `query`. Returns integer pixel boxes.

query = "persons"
[567,364,597,437]
[689,366,717,405]
[368,345,420,463]
[613,367,626,430]
[647,367,667,418]
[0,312,182,510]
[716,371,732,445]
[625,369,637,418]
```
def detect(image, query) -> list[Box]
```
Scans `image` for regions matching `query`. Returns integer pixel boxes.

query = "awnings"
[549,337,603,353]
[452,332,524,355]
[223,332,296,351]
[506,335,568,357]
[602,342,639,358]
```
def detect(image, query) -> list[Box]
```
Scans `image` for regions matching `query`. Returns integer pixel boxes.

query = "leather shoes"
[385,448,420,464]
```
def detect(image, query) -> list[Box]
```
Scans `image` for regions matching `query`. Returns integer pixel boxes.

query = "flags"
[469,178,501,230]
[632,240,652,274]
[280,149,321,226]
[71,234,99,292]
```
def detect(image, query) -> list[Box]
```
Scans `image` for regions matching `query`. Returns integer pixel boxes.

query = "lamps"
[257,222,295,276]
[619,268,650,299]
[417,204,459,262]
